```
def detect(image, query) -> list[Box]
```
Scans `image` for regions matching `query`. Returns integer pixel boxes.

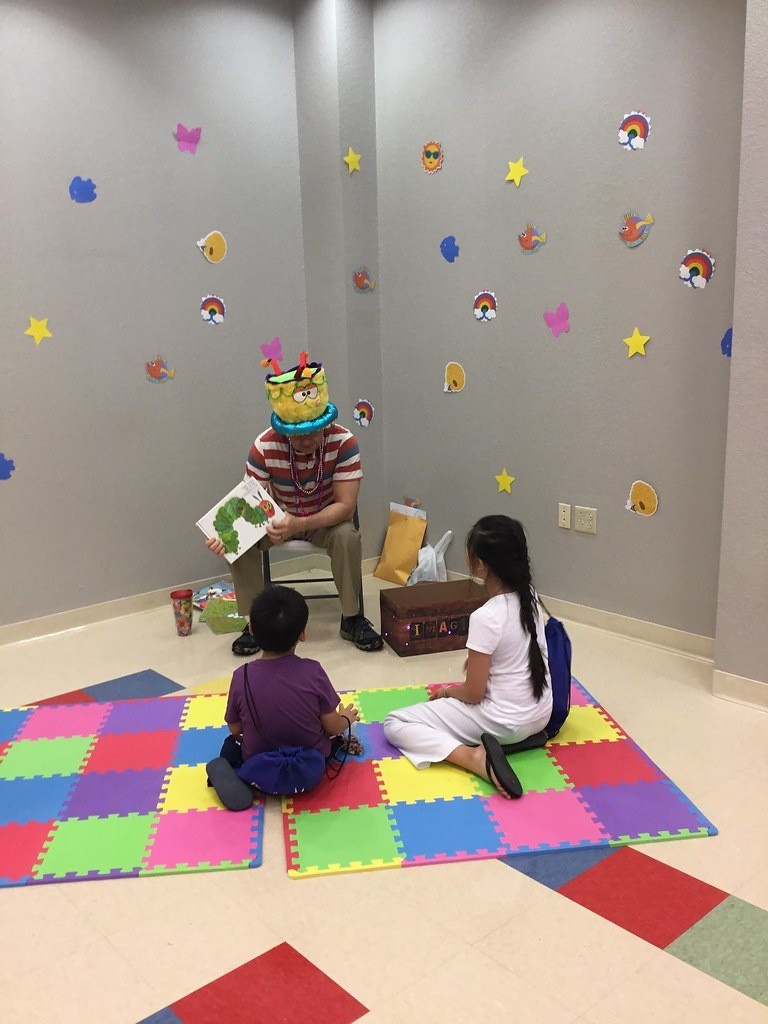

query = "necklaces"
[288,433,325,497]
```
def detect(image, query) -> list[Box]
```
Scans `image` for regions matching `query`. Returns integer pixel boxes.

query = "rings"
[280,533,285,539]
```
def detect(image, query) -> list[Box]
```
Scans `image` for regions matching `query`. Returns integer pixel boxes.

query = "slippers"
[481,733,522,798]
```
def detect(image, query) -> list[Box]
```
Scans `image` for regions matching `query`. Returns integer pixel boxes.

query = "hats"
[261,351,338,435]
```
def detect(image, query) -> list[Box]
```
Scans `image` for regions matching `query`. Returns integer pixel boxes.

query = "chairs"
[263,506,363,616]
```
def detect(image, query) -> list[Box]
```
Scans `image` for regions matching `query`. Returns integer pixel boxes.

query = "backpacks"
[236,664,351,794]
[538,597,571,739]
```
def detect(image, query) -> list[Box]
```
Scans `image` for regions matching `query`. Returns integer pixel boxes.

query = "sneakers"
[232,623,260,656]
[340,611,384,650]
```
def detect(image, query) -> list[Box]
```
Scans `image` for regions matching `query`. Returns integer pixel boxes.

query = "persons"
[206,584,360,810]
[383,514,554,800]
[205,397,381,656]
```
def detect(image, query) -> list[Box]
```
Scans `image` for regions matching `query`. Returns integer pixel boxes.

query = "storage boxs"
[378,579,487,658]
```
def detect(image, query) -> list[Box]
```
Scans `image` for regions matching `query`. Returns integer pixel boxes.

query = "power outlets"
[558,503,598,534]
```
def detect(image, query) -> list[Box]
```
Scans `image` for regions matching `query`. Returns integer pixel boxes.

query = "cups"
[171,589,193,637]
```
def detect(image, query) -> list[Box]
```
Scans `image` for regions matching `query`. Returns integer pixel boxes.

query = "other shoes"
[206,758,253,811]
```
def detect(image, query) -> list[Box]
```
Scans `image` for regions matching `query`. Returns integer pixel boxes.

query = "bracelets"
[443,685,453,698]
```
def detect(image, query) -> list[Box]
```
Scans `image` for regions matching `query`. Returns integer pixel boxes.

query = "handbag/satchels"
[407,530,453,586]
